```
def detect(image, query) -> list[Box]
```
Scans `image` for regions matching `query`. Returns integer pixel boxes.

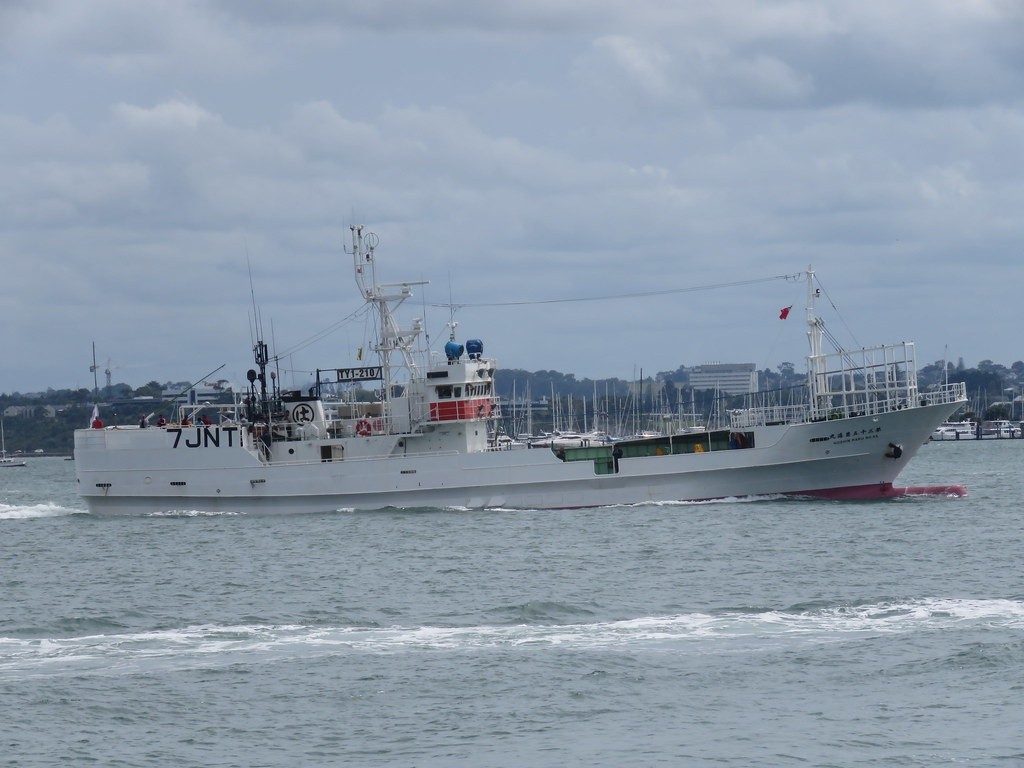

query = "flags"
[779,305,793,319]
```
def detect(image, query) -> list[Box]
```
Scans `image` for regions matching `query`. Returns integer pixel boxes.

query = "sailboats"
[0,419,26,467]
[493,359,1023,464]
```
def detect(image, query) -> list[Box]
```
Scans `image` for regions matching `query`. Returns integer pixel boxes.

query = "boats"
[72,221,970,514]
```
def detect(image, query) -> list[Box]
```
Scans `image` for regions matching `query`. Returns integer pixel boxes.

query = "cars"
[34,448,44,453]
[15,450,22,453]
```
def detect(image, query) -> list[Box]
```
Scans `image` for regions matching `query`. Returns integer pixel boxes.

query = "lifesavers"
[356,420,372,437]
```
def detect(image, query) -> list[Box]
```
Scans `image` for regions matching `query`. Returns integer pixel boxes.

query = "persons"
[92,416,103,428]
[157,415,166,427]
[181,414,192,425]
[194,418,205,425]
[138,415,148,428]
[236,414,250,431]
[260,428,271,461]
[281,410,293,440]
[223,415,234,424]
[201,415,211,425]
